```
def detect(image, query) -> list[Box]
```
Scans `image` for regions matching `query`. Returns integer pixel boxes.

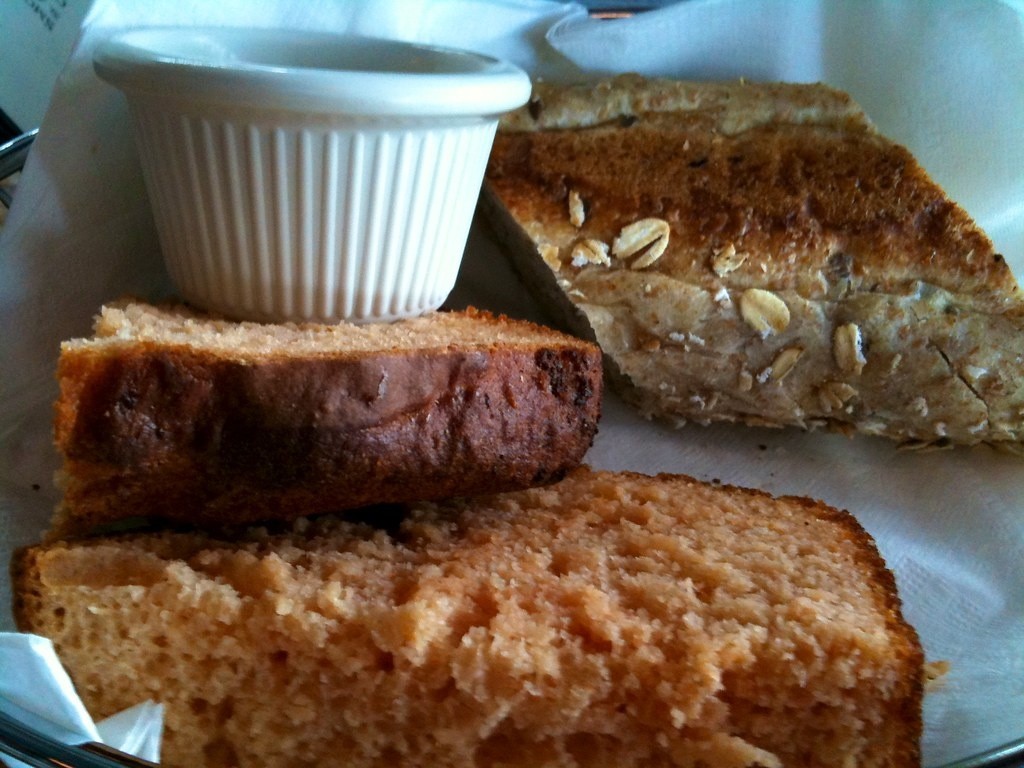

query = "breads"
[14,471,925,768]
[53,301,601,525]
[479,83,1024,447]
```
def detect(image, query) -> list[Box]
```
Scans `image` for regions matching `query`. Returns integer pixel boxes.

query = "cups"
[93,25,532,325]
